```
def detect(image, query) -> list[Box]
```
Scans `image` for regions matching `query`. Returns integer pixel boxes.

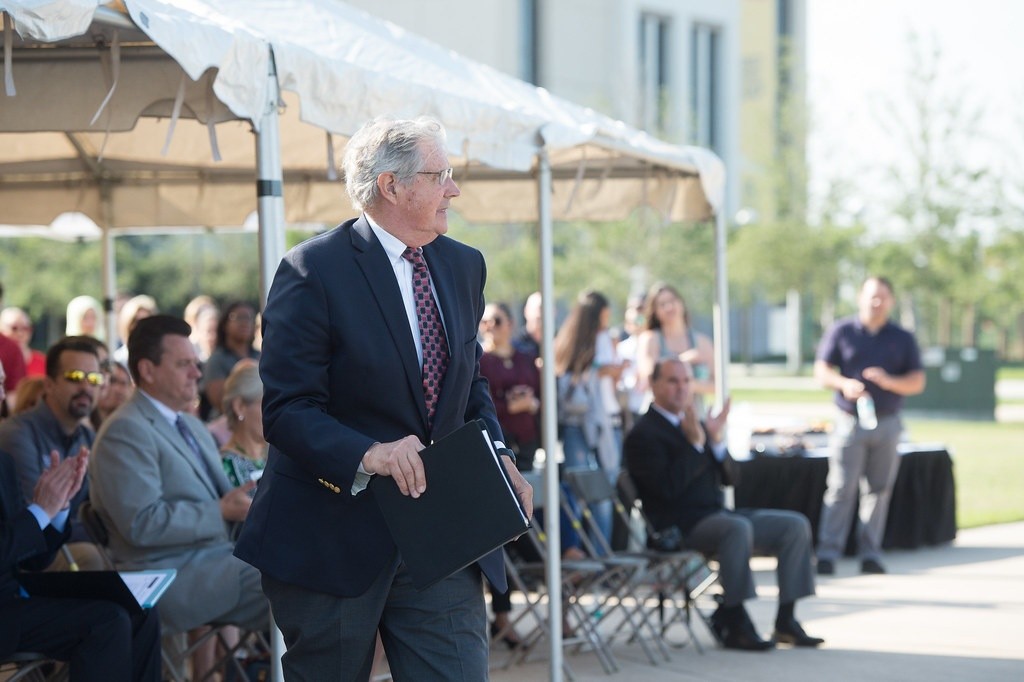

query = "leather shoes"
[711,607,774,650]
[770,620,824,650]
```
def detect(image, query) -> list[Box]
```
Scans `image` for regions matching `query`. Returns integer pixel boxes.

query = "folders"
[368,418,533,594]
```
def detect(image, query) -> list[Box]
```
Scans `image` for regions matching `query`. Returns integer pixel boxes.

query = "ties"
[401,247,450,432]
[176,415,209,474]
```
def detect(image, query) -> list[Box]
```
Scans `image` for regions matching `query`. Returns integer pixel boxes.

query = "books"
[368,418,533,589]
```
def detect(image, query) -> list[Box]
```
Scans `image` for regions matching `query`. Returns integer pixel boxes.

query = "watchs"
[497,448,516,465]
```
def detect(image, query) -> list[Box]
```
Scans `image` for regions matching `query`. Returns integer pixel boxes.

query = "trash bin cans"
[898,347,996,423]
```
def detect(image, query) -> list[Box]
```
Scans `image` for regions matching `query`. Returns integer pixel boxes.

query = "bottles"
[856,393,878,430]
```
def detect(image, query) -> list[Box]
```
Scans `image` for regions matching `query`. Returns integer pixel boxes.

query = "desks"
[719,433,956,552]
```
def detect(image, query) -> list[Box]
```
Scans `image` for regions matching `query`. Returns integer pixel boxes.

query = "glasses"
[58,369,105,387]
[480,315,505,326]
[418,168,454,184]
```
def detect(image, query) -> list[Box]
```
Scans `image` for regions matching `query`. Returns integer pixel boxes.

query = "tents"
[0,0,728,681]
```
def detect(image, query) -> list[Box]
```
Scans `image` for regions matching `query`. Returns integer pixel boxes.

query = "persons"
[816,277,925,576]
[88,316,270,637]
[480,283,714,651]
[0,283,269,682]
[230,118,535,682]
[622,358,826,649]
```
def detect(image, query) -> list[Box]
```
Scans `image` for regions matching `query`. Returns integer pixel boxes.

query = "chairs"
[490,474,718,682]
[2,621,283,682]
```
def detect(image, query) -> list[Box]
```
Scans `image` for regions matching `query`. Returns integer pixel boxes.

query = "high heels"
[490,622,528,650]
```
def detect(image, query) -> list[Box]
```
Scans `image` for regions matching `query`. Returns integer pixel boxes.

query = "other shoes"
[860,560,885,575]
[818,559,834,574]
